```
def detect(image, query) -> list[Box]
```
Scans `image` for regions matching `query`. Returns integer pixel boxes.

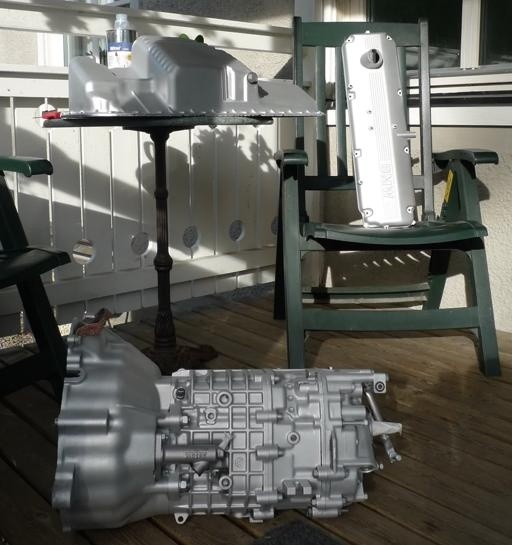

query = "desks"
[41,110,270,373]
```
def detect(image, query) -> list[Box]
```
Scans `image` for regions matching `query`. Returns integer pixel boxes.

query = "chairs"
[270,16,503,386]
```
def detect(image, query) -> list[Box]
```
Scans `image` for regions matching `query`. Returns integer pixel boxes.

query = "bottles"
[112,12,131,30]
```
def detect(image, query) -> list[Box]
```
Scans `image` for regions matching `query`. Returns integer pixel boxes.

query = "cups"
[107,30,137,45]
